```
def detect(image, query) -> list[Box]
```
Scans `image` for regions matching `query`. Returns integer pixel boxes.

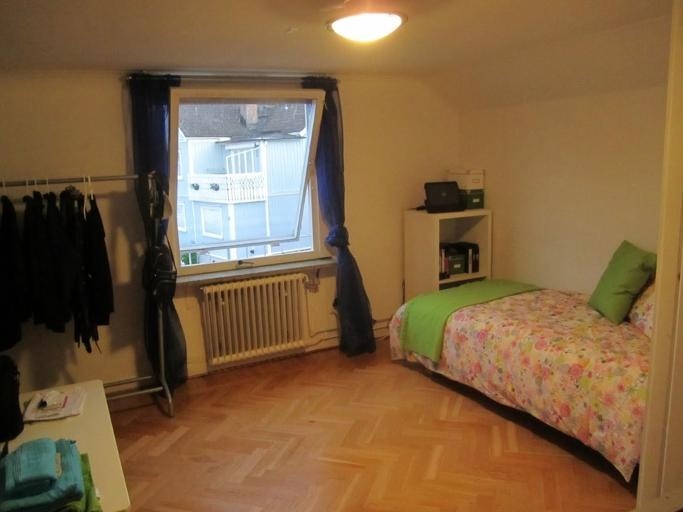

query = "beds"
[389,278,653,441]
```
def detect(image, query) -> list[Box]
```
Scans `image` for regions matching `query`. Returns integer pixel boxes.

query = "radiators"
[199,273,313,367]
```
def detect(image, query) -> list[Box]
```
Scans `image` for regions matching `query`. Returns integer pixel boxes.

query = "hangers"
[0,177,96,221]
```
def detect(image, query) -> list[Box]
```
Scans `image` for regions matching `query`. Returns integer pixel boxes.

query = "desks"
[8,378,133,512]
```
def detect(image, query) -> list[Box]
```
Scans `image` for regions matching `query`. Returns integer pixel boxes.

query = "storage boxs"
[448,168,484,209]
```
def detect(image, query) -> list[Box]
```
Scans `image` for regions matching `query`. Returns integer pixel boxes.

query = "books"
[21,388,86,421]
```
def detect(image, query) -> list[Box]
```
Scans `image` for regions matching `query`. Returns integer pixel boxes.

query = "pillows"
[588,241,656,341]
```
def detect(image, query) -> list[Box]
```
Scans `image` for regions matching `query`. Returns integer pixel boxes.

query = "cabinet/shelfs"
[405,211,492,302]
